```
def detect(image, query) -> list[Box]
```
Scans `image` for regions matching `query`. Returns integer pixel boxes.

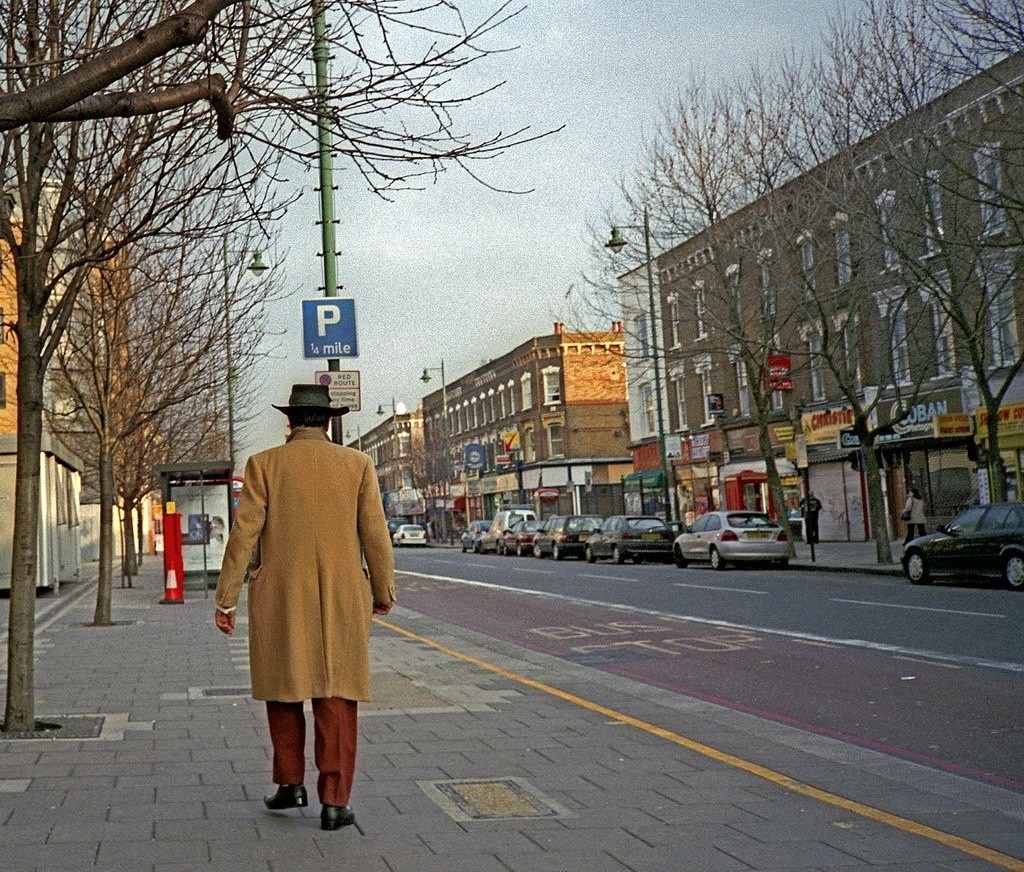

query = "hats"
[272,383,349,417]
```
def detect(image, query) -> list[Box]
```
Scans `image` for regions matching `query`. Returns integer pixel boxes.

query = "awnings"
[624,469,662,488]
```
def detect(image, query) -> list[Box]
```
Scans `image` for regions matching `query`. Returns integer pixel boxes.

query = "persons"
[214,384,397,831]
[800,491,822,544]
[902,488,927,547]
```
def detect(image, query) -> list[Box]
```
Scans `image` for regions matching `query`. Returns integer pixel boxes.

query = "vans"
[531,512,608,560]
[480,508,539,555]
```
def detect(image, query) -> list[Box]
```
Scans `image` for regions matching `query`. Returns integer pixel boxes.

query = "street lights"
[420,359,455,548]
[375,398,401,502]
[604,205,673,527]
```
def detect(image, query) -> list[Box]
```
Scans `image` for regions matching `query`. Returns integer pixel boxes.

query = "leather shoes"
[265,784,308,809]
[320,803,355,830]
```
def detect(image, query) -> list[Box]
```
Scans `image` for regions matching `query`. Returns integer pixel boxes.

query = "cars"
[385,519,428,548]
[501,520,547,559]
[582,515,676,565]
[669,509,792,570]
[899,502,1024,591]
[460,518,494,553]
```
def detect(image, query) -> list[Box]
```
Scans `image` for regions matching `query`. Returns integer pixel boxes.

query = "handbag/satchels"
[901,511,911,521]
